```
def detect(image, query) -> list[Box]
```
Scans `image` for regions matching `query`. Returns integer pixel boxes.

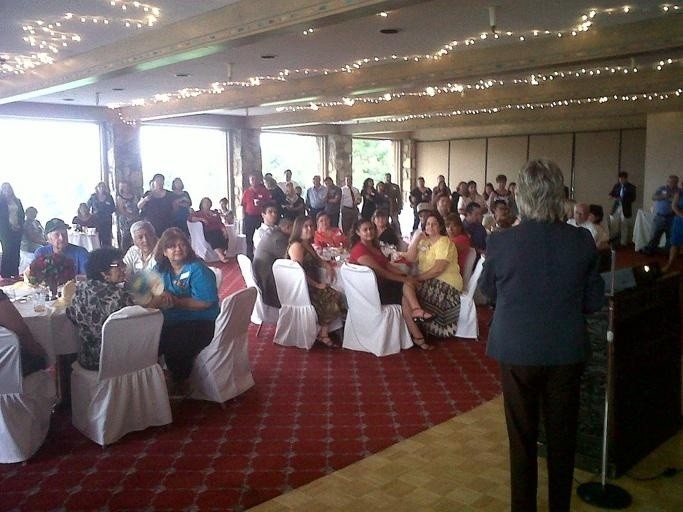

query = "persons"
[479,158,603,510]
[644,174,683,272]
[563,170,636,253]
[0,169,519,376]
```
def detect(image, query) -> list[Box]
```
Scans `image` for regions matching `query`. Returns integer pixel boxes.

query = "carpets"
[2,239,683,512]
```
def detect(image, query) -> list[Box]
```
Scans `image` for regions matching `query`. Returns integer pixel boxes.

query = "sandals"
[411,308,437,322]
[412,336,436,352]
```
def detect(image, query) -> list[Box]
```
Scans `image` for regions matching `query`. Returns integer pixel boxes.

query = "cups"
[254,199,260,207]
[3,282,64,313]
[70,225,97,235]
[312,241,350,264]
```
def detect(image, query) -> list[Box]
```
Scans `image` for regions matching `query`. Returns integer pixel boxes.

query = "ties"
[346,184,356,208]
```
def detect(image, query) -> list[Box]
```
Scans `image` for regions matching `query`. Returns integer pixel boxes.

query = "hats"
[416,202,431,213]
[43,218,71,234]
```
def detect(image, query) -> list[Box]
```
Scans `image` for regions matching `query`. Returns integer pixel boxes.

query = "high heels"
[315,334,338,350]
